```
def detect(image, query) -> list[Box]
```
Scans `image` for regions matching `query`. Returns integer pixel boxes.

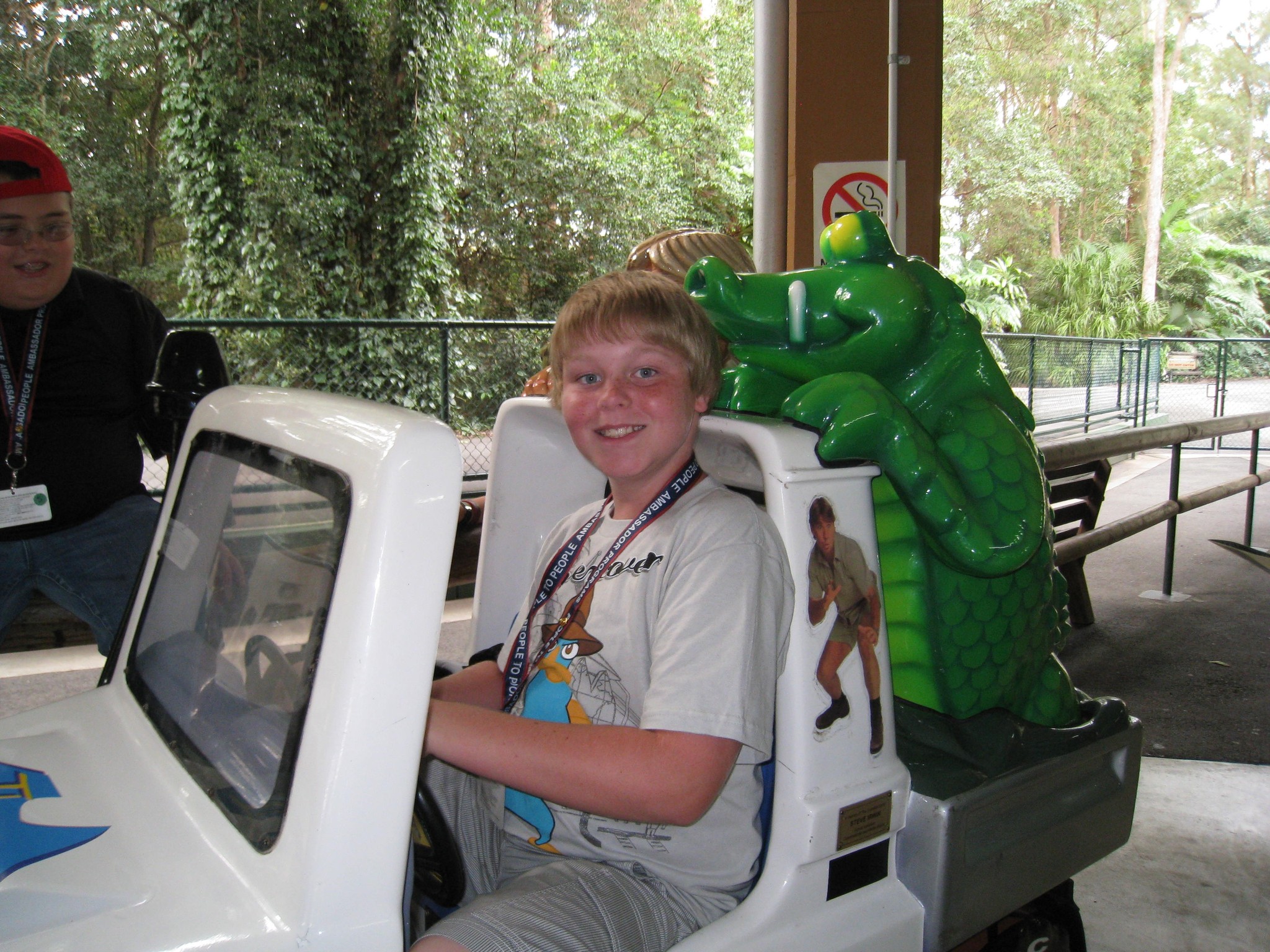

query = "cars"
[0,209,1142,952]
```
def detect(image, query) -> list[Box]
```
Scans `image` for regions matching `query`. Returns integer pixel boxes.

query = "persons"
[808,496,882,757]
[0,123,171,657]
[263,269,795,952]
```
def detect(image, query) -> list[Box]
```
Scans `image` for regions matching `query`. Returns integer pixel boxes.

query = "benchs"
[1042,458,1112,629]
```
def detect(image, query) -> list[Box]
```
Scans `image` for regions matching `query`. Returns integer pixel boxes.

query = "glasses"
[0,216,80,246]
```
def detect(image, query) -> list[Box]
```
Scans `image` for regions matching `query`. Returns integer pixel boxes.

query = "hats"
[0,124,72,200]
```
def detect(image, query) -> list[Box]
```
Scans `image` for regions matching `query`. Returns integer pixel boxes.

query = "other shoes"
[870,718,884,755]
[816,689,850,730]
